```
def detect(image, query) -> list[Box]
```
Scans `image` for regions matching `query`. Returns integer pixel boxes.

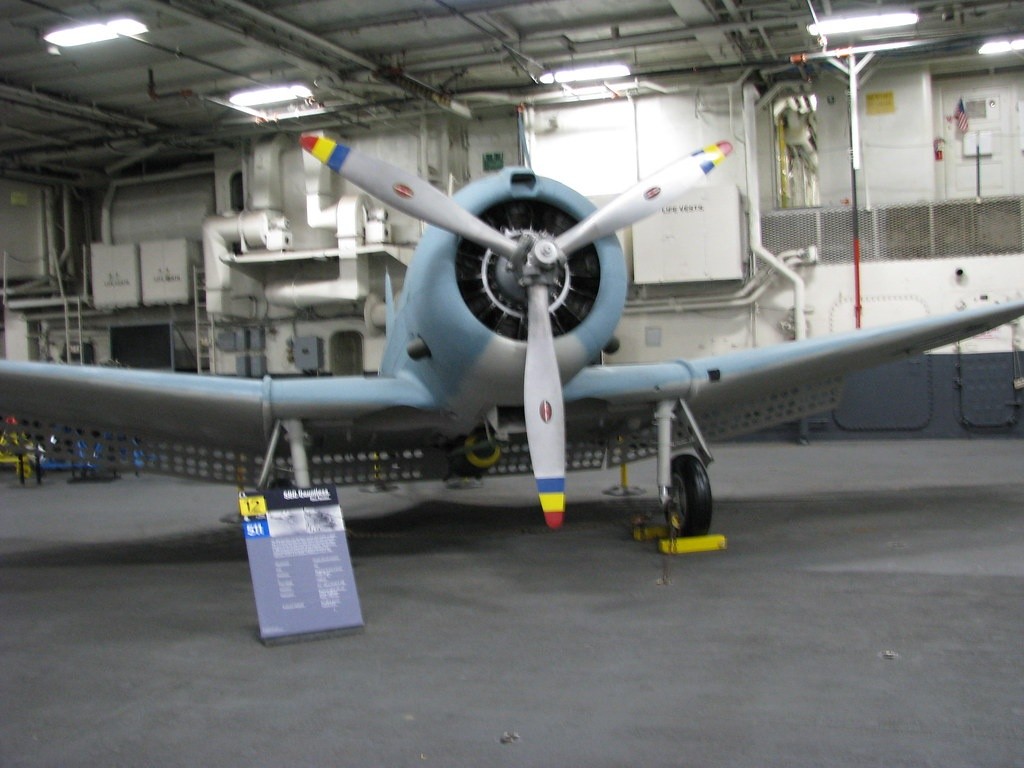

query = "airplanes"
[1,131,1022,551]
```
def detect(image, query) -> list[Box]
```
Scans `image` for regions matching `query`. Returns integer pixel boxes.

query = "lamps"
[43,12,148,48]
[228,81,313,106]
[538,60,631,84]
[807,5,918,35]
[977,35,1024,55]
